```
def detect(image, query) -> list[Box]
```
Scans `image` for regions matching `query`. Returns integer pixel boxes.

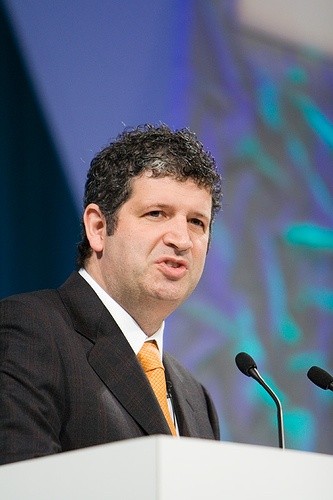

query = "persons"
[0,122,222,466]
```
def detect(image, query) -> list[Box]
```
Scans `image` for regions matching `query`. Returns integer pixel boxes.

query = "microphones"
[235,352,284,448]
[307,366,333,391]
[165,380,172,394]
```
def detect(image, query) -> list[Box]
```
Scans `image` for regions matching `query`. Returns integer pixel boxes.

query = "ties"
[137,340,179,437]
